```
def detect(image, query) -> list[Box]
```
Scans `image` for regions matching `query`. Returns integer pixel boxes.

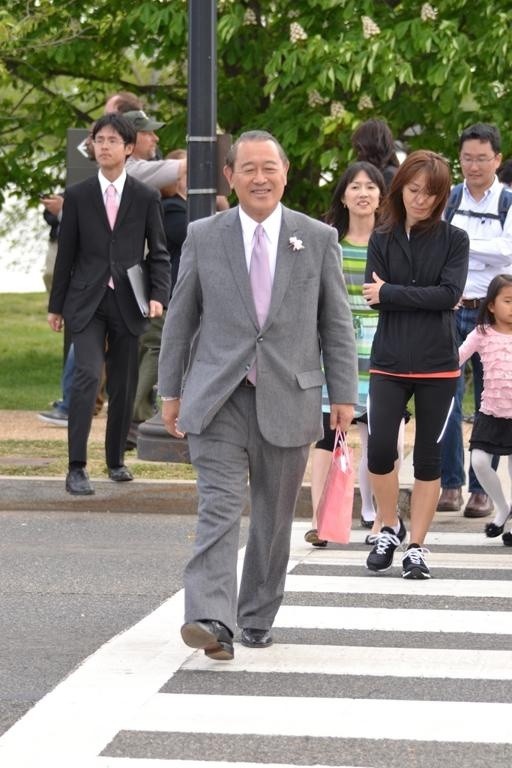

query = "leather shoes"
[304,528,327,546]
[65,468,94,494]
[486,504,512,545]
[108,466,134,481]
[242,627,272,648]
[359,515,374,527]
[464,490,494,518]
[437,488,463,512]
[181,620,234,661]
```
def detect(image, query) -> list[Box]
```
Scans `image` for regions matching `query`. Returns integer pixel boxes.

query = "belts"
[463,299,481,309]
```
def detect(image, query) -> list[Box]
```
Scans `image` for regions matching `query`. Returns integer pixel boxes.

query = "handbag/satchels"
[317,447,356,544]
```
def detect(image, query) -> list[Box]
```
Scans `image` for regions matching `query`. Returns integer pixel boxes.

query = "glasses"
[462,153,496,165]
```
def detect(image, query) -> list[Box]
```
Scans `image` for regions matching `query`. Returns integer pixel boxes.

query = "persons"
[49,115,171,494]
[304,121,510,545]
[361,151,470,578]
[158,131,359,660]
[37,90,229,451]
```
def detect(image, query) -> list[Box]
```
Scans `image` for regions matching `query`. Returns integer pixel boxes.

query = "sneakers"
[400,543,432,579]
[365,516,407,572]
[39,411,69,427]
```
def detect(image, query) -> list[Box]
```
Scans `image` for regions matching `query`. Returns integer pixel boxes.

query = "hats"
[122,110,166,131]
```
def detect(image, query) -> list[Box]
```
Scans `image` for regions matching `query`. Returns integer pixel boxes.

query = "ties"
[247,224,275,386]
[104,185,117,291]
